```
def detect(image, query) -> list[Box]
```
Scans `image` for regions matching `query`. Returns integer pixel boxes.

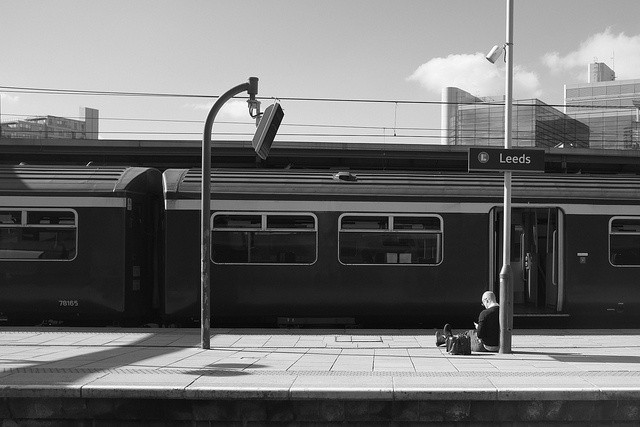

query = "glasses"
[481,298,487,306]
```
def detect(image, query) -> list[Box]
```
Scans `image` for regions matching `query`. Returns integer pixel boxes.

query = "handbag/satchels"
[447,333,471,355]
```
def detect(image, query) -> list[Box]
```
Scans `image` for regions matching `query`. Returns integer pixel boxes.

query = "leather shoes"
[436,330,445,346]
[444,324,453,338]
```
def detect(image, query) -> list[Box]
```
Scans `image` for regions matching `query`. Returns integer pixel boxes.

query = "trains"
[0,138,639,330]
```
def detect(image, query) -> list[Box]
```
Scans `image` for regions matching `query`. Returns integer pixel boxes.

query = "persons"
[436,290,500,352]
